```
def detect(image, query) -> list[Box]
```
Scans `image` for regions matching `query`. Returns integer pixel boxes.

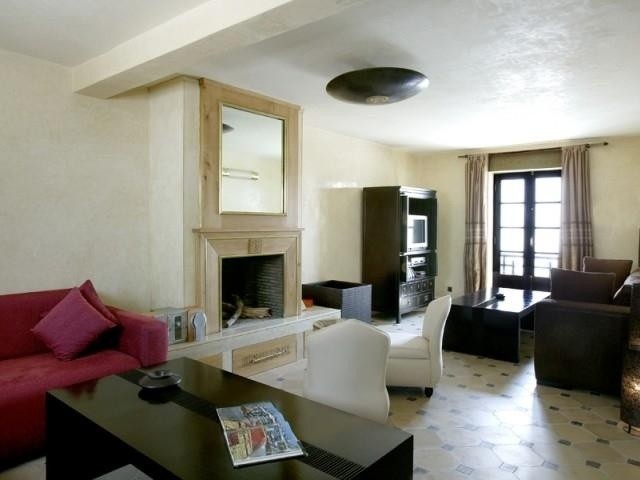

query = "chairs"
[297,319,391,423]
[386,295,453,398]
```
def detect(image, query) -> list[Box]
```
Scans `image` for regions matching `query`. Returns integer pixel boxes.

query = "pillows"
[550,268,617,303]
[583,256,632,290]
[30,280,117,361]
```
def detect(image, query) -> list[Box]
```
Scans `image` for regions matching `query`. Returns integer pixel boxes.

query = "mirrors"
[218,102,288,216]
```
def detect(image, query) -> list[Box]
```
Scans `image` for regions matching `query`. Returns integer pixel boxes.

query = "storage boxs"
[303,280,373,324]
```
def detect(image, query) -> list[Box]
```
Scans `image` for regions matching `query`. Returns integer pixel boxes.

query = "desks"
[442,285,552,364]
[46,358,413,480]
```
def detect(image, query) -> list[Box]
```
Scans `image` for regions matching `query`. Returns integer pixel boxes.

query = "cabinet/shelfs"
[153,306,342,394]
[363,186,438,324]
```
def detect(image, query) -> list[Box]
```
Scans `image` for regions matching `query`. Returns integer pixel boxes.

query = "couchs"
[535,259,640,396]
[0,288,168,471]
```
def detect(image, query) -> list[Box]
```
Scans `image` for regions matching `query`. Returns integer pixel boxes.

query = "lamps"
[326,67,429,106]
[222,168,259,180]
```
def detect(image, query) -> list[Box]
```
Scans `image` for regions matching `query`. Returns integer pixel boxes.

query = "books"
[215,400,309,466]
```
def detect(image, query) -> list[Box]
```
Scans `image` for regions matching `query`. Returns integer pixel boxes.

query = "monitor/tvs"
[406,214,429,249]
